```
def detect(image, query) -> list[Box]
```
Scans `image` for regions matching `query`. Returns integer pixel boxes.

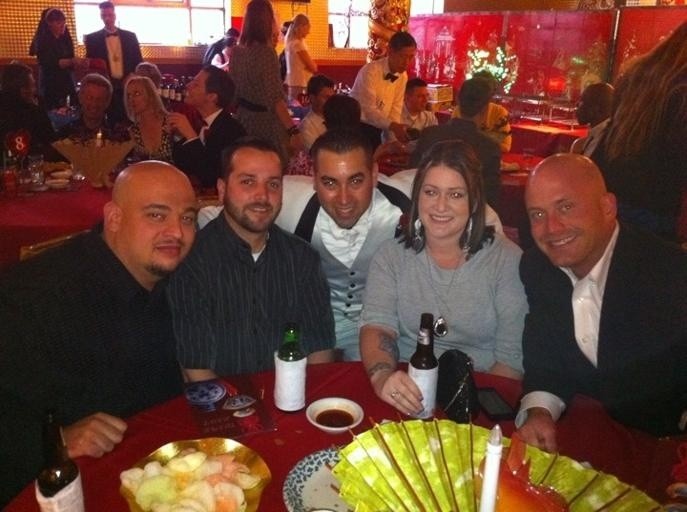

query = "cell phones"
[478,387,515,421]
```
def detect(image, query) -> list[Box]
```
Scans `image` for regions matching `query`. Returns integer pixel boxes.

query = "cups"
[25,151,44,185]
[165,117,182,144]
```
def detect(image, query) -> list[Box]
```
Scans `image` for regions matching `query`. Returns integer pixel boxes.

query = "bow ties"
[385,73,398,83]
[104,30,118,37]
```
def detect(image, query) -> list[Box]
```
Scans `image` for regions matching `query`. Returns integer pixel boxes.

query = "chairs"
[20,229,91,263]
[198,195,220,208]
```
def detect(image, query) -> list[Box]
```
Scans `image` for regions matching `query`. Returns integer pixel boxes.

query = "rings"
[391,391,398,397]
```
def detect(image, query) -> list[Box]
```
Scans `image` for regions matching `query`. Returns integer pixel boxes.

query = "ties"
[199,121,209,146]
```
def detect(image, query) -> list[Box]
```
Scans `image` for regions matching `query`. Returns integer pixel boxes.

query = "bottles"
[406,312,439,420]
[271,321,310,413]
[30,396,86,511]
[3,159,19,195]
[160,77,192,103]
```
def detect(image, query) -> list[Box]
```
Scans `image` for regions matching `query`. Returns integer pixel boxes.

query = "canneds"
[3,170,17,198]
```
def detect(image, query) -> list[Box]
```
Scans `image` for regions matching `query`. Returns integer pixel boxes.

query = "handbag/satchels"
[437,347,482,424]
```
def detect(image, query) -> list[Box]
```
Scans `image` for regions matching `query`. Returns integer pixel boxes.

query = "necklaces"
[422,247,464,338]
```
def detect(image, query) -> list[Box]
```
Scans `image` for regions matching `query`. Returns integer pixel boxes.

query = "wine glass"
[333,80,350,94]
[522,147,537,173]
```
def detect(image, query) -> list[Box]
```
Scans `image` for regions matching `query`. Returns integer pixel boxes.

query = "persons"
[1,1,437,186]
[512,152,687,457]
[0,159,198,507]
[272,125,414,364]
[359,138,530,416]
[569,83,615,160]
[155,135,337,384]
[590,21,687,244]
[449,78,512,153]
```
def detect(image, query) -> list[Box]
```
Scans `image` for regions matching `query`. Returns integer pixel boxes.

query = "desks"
[500,149,546,224]
[0,162,217,265]
[0,360,687,511]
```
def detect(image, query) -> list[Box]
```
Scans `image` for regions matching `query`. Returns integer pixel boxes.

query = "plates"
[44,167,72,190]
[118,436,272,510]
[304,397,366,434]
[386,156,411,167]
[284,446,354,510]
